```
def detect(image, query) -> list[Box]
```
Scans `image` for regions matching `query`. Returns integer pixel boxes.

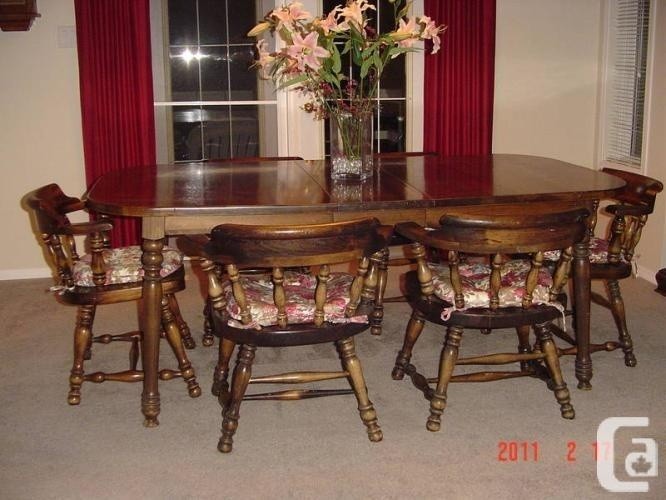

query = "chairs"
[533,168,663,367]
[208,156,304,162]
[394,205,590,432]
[20,181,201,405]
[373,151,439,159]
[174,215,391,453]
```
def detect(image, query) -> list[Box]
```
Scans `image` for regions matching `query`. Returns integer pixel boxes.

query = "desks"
[83,152,627,429]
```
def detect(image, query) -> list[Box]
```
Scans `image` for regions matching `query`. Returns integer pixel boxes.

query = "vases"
[329,112,375,180]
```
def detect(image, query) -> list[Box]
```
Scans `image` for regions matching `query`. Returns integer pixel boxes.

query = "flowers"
[245,0,449,159]
